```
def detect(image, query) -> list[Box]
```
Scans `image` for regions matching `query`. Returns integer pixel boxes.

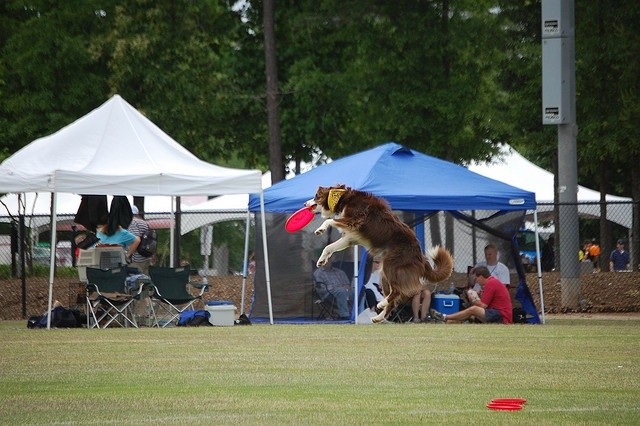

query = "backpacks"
[137,221,158,258]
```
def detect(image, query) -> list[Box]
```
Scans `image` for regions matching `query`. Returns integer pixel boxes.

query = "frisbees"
[491,397,527,404]
[285,206,315,233]
[486,403,523,410]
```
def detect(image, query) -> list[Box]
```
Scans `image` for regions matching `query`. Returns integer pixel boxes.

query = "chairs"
[310,260,365,321]
[148,265,210,328]
[85,267,144,328]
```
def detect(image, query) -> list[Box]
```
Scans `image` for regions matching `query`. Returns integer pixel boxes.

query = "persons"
[411,287,435,324]
[430,265,513,326]
[364,255,385,312]
[579,240,601,274]
[96,213,142,259]
[126,205,153,273]
[313,256,354,320]
[469,244,511,305]
[609,239,630,272]
[519,236,556,271]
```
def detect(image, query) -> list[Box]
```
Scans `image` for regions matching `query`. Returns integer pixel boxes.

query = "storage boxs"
[433,294,461,314]
[205,303,237,326]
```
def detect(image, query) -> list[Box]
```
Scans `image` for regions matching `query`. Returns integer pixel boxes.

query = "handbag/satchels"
[512,308,527,323]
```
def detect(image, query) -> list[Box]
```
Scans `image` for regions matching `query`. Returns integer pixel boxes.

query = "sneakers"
[429,308,445,322]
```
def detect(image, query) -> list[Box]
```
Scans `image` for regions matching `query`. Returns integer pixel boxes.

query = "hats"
[618,240,624,244]
[373,256,382,261]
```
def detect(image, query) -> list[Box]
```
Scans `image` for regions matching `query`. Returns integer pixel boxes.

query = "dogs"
[303,182,455,324]
